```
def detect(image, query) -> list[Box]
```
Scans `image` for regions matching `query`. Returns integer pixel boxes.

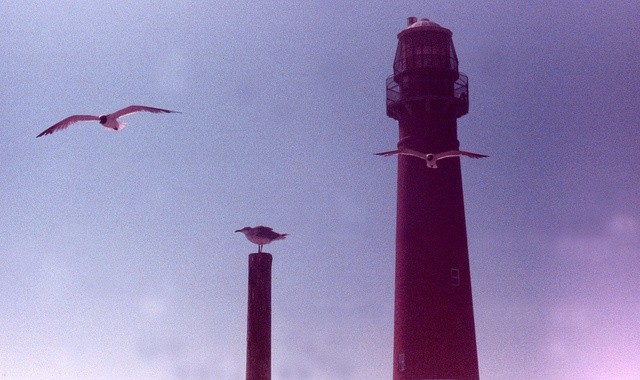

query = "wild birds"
[371,146,493,170]
[234,225,290,253]
[36,105,183,139]
